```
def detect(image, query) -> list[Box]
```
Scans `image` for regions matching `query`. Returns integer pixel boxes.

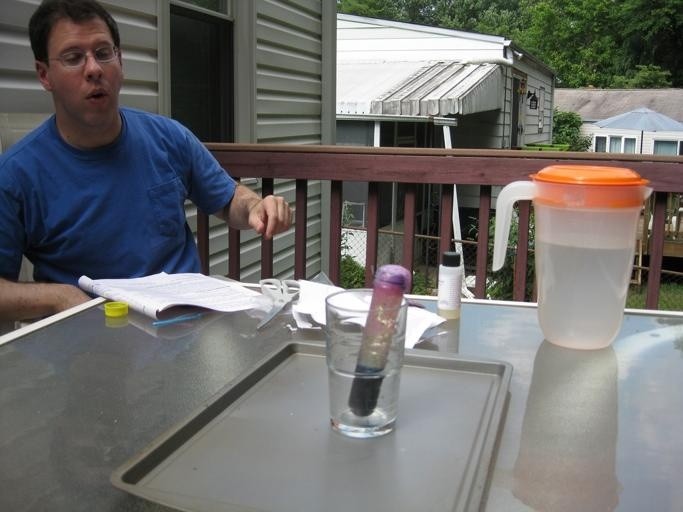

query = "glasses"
[43,45,119,68]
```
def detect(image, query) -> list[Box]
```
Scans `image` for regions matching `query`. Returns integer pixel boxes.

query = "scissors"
[256,278,300,329]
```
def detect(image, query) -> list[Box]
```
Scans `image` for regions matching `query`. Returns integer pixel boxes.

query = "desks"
[0,281,682,512]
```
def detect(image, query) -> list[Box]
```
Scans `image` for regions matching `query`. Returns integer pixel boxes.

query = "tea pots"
[491,165,655,351]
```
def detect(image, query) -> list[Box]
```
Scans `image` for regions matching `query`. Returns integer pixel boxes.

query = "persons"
[0,0,294,334]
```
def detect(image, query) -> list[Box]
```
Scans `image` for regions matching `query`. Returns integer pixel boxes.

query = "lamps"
[527,87,539,110]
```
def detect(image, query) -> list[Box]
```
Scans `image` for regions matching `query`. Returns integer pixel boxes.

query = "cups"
[325,288,408,439]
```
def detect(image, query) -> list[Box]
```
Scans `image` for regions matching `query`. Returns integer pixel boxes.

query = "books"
[75,270,270,319]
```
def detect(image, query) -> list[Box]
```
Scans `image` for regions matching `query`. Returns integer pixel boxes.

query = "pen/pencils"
[151,313,204,325]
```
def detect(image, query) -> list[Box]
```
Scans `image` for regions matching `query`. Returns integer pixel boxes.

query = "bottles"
[436,252,463,320]
[347,265,411,417]
